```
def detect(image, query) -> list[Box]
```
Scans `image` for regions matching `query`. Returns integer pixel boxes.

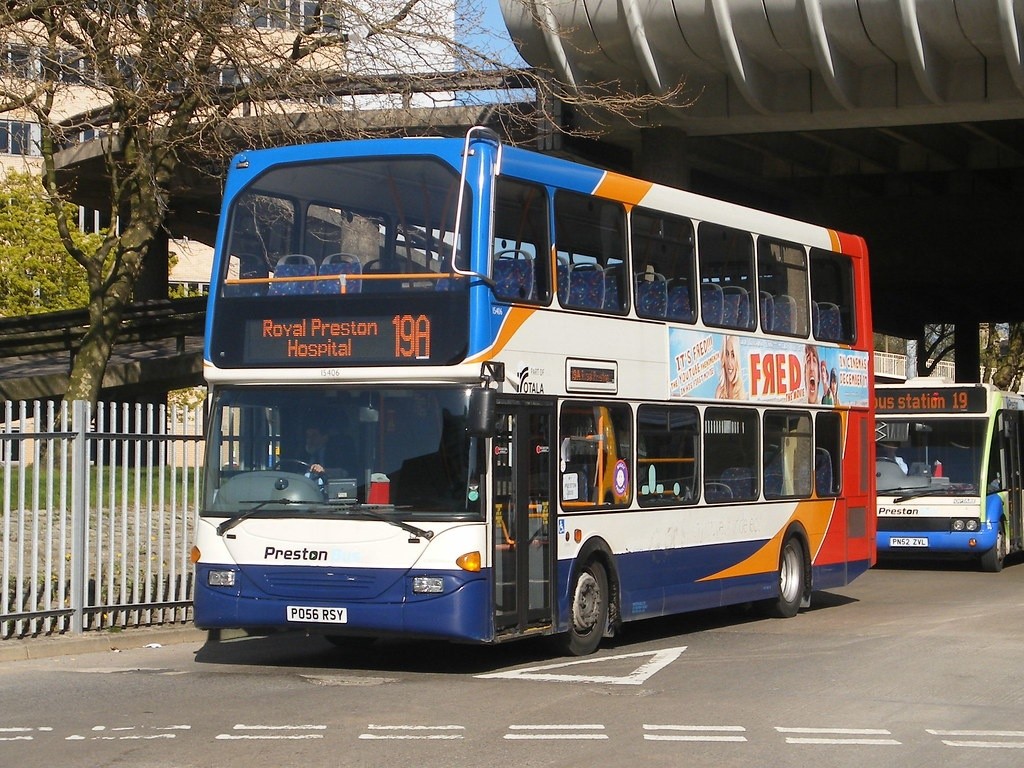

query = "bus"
[189,133,879,658]
[869,374,1024,572]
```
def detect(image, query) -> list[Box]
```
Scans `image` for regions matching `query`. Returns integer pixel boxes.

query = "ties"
[314,454,321,464]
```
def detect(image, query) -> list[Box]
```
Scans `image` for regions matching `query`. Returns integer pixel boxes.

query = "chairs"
[435,249,842,343]
[705,444,832,503]
[268,253,402,294]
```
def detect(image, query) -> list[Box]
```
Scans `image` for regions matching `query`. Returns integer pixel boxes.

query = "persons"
[881,441,908,475]
[715,335,745,400]
[290,422,351,494]
[804,345,840,407]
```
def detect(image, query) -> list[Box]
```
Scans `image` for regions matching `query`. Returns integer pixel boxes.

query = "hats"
[821,361,828,382]
